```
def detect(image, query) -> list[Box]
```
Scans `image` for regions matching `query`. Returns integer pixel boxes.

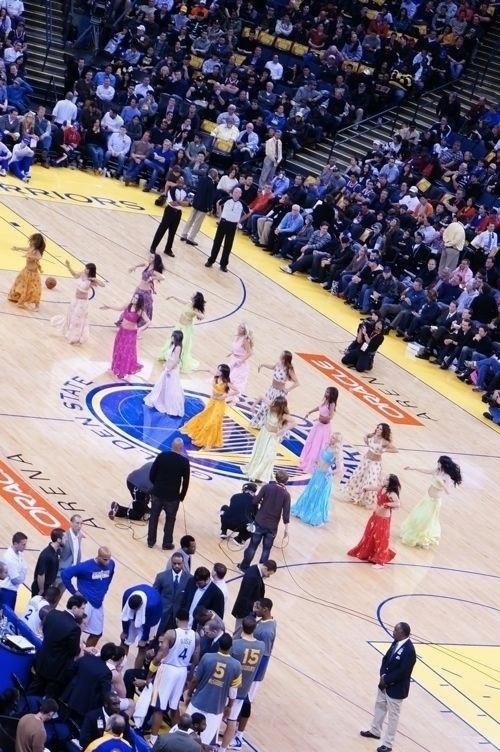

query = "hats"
[409,186,418,193]
[137,25,146,31]
[22,135,30,144]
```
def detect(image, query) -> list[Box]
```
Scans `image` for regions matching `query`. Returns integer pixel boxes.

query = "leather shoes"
[150,249,155,253]
[221,265,227,272]
[164,249,175,257]
[416,345,485,392]
[181,237,198,245]
[340,291,370,315]
[360,730,380,739]
[205,262,212,267]
[390,326,414,342]
[377,745,392,752]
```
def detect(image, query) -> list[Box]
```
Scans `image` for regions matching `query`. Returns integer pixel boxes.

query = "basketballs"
[46,277,57,288]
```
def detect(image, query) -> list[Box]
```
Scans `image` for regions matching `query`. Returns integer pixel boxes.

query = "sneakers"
[162,544,174,550]
[108,502,118,520]
[97,167,130,185]
[280,265,292,274]
[23,172,31,182]
[220,530,227,538]
[227,736,243,750]
[308,275,332,290]
[233,536,244,546]
[143,187,149,192]
[0,166,7,176]
[244,229,284,257]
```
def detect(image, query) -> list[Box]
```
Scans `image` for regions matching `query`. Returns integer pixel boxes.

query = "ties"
[173,575,178,591]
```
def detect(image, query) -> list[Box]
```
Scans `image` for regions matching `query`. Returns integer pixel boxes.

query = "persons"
[1,0,500,751]
